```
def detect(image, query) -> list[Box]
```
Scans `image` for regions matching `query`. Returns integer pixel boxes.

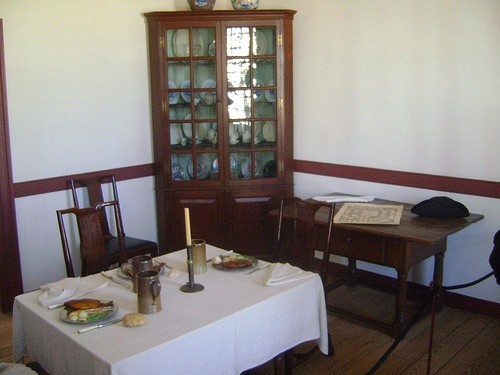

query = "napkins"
[38,276,80,307]
[266,261,318,285]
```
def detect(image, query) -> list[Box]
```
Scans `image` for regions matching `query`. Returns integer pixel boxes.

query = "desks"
[265,193,485,340]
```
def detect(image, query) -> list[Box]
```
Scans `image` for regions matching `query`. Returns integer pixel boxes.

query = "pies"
[123,313,148,327]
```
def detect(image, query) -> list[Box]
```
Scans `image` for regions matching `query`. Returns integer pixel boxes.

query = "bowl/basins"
[194,99,200,105]
[187,0,216,10]
[231,0,260,10]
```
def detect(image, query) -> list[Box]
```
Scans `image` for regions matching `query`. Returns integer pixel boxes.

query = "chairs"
[68,172,159,271]
[55,200,129,279]
[269,195,335,375]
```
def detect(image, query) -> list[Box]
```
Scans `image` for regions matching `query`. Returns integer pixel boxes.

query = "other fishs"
[216,260,252,268]
[64,299,113,310]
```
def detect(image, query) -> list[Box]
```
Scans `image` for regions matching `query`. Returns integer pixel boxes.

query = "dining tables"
[12,244,328,375]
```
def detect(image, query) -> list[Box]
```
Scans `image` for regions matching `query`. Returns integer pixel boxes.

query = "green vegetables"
[87,310,113,320]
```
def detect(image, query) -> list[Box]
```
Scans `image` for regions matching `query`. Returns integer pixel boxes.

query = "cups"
[242,132,251,143]
[191,239,208,274]
[131,255,153,293]
[180,138,186,146]
[205,97,213,104]
[135,270,162,314]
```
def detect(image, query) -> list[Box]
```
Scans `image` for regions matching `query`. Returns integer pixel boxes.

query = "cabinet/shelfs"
[143,8,297,257]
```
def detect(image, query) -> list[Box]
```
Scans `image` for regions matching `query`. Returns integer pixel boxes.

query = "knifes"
[78,318,122,333]
[248,263,270,274]
[100,271,130,289]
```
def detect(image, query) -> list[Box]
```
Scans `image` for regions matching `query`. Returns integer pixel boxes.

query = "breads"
[69,311,89,321]
[212,257,222,265]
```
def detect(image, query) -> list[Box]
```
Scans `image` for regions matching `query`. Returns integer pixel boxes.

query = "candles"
[184,206,191,246]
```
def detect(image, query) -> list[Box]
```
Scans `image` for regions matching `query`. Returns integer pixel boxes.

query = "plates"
[117,260,165,280]
[168,77,277,104]
[211,254,258,272]
[170,112,276,146]
[58,299,118,324]
[172,154,275,180]
[172,28,268,63]
